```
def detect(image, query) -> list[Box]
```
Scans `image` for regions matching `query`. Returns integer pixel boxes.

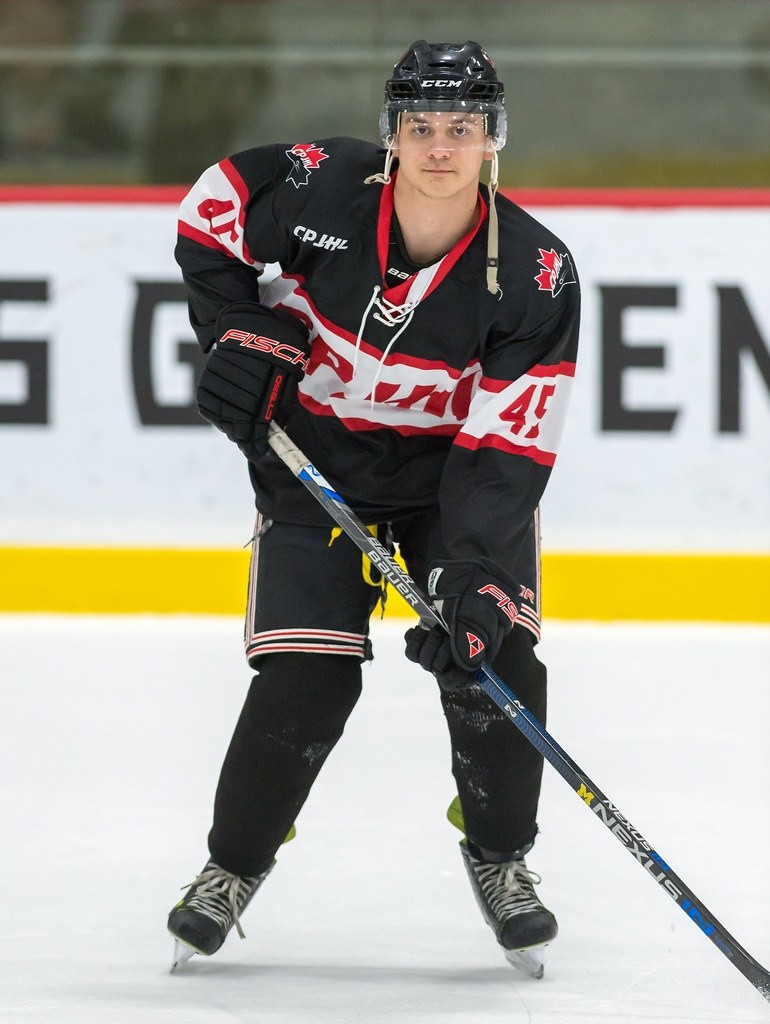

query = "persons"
[167,40,582,956]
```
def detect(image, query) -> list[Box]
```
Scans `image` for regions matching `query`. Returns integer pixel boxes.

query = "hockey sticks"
[265,416,770,1003]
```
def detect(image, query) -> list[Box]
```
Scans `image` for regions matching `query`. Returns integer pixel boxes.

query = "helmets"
[384,39,505,139]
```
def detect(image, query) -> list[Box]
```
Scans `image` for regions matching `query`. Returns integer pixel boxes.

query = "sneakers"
[167,825,295,973]
[445,795,557,978]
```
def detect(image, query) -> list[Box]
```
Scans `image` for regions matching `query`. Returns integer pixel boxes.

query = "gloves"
[195,301,313,462]
[402,558,522,692]
